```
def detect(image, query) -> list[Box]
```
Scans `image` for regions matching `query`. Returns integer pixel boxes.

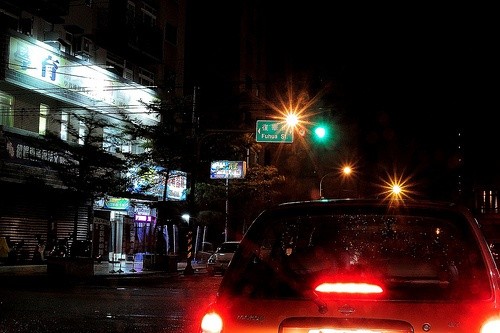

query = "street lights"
[320,166,352,199]
[375,184,401,199]
[181,213,203,258]
[246,106,304,168]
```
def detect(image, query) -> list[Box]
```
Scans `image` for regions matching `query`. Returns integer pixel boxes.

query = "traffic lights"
[314,125,329,140]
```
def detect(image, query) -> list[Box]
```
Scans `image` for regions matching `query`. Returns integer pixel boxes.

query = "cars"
[198,198,500,333]
[205,241,241,275]
